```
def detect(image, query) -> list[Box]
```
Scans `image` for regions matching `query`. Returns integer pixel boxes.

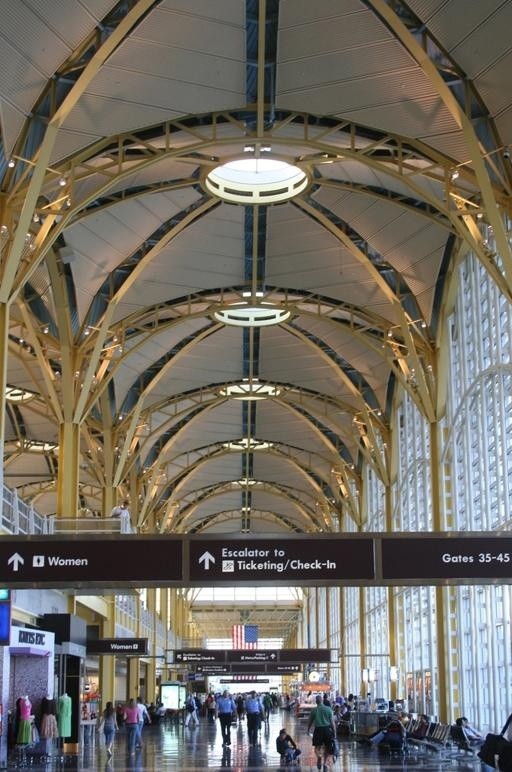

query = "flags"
[229,622,259,650]
[231,673,257,681]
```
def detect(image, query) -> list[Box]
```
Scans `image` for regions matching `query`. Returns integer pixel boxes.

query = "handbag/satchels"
[330,740,339,756]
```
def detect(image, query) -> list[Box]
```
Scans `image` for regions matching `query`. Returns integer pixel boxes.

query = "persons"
[322,691,430,752]
[122,696,140,756]
[94,700,119,757]
[125,747,142,771]
[455,716,488,764]
[36,695,59,757]
[181,726,218,770]
[220,745,233,771]
[12,694,33,749]
[55,691,72,738]
[215,689,237,747]
[461,715,481,736]
[240,689,267,747]
[245,721,271,772]
[116,696,165,731]
[110,499,135,535]
[305,694,337,771]
[102,756,114,772]
[274,727,303,762]
[180,688,290,727]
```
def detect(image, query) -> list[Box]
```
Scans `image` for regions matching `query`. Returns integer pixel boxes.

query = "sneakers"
[316,756,331,770]
[185,716,269,747]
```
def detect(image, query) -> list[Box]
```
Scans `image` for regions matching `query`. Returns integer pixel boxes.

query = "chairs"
[406,718,487,761]
